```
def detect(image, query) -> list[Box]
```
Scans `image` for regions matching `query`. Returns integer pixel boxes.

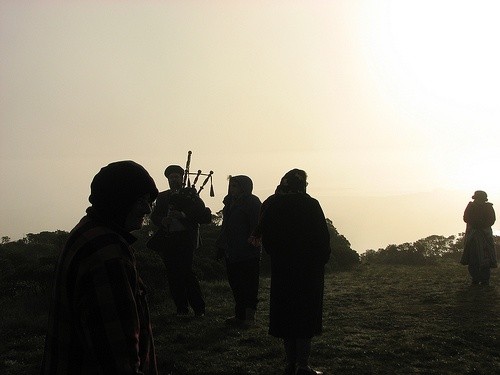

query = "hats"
[165,166,184,178]
[472,191,488,201]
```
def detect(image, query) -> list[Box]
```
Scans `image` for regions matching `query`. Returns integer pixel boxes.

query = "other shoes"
[285,365,295,375]
[195,313,205,321]
[177,310,189,318]
[297,365,323,375]
[226,315,253,326]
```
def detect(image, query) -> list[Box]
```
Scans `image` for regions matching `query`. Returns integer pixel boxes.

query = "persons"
[41,160,158,375]
[148,164,213,319]
[215,175,262,331]
[460,191,497,286]
[258,169,331,374]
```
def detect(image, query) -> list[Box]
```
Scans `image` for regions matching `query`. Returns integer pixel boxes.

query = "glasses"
[306,181,308,186]
